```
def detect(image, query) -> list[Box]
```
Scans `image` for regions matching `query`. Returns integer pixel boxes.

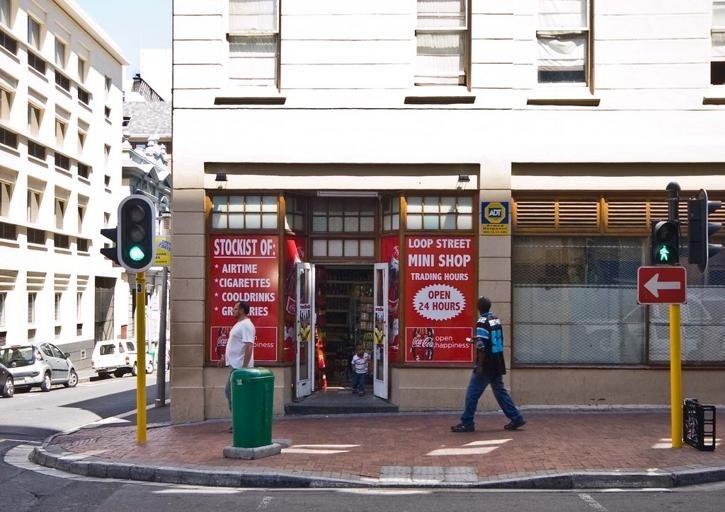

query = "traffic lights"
[100,194,155,273]
[650,189,722,273]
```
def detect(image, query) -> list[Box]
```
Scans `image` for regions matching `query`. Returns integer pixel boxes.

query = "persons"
[225,302,256,433]
[451,296,526,432]
[351,344,373,398]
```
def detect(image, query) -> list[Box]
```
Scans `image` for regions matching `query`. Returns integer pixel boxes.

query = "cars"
[92,339,153,378]
[0,341,78,397]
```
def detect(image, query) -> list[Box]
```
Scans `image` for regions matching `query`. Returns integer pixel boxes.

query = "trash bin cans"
[230,368,276,447]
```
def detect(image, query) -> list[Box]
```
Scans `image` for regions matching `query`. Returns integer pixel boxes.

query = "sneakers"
[352,385,365,396]
[504,418,526,431]
[450,422,475,433]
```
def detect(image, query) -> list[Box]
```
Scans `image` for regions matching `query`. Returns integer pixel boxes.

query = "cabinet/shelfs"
[324,280,373,377]
